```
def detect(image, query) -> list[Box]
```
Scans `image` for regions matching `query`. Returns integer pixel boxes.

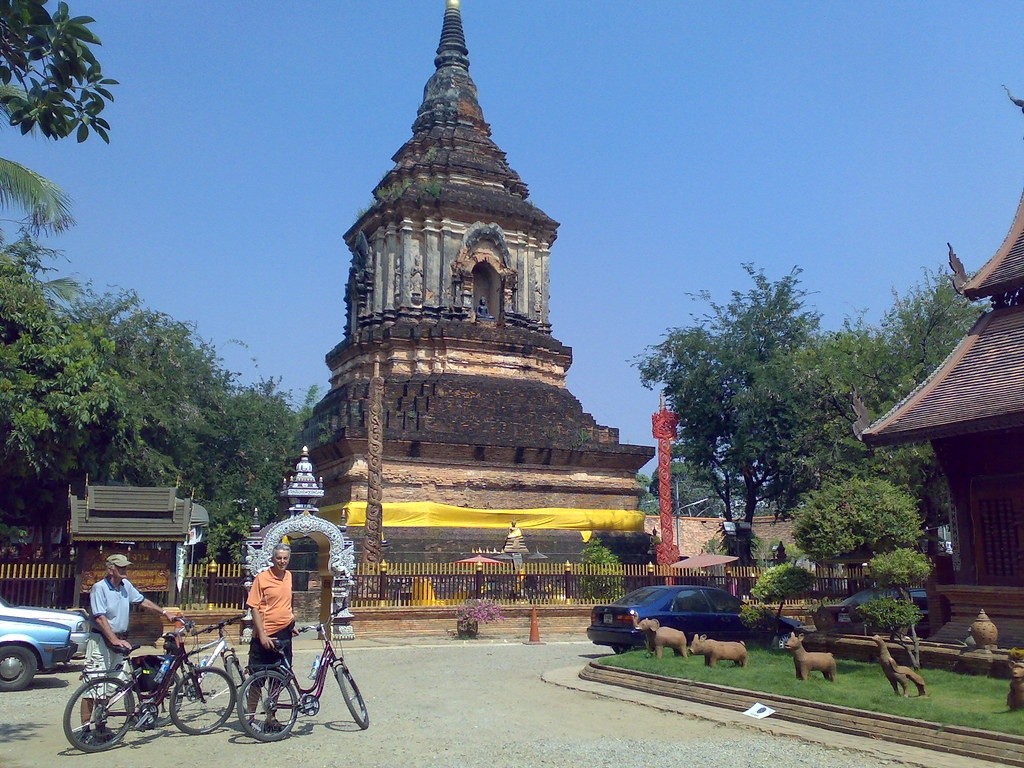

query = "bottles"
[310,654,321,679]
[200,655,208,666]
[153,656,173,684]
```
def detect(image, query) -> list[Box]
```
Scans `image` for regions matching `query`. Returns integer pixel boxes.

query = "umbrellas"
[456,554,503,564]
[670,549,739,570]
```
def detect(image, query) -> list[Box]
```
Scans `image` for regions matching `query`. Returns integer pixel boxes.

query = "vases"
[457,618,479,641]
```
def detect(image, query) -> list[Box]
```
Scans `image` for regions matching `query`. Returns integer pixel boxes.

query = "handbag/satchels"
[91,628,129,648]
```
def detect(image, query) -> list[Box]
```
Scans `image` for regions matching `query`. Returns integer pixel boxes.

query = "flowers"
[455,596,506,634]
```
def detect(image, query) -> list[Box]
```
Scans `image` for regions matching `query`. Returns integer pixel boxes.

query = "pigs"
[690,633,747,669]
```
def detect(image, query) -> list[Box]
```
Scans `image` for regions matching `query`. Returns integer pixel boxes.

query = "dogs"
[784,632,836,682]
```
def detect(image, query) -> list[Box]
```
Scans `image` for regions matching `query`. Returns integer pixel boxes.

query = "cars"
[0,615,78,690]
[815,587,951,640]
[587,585,799,657]
[0,594,93,659]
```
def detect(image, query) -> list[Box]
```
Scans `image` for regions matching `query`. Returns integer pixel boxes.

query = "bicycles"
[123,611,249,728]
[62,614,237,752]
[234,607,369,741]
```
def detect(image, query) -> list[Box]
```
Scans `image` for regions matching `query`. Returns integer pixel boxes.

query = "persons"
[476,296,495,319]
[247,543,300,735]
[647,526,661,554]
[80,555,178,747]
[505,521,523,540]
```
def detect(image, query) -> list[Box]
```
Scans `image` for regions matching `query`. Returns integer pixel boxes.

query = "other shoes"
[80,732,93,745]
[245,720,264,736]
[264,719,292,733]
[94,729,116,739]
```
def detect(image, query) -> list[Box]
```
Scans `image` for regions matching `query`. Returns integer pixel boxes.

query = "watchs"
[162,610,167,616]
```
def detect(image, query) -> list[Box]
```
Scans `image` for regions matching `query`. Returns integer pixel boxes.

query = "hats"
[106,554,134,567]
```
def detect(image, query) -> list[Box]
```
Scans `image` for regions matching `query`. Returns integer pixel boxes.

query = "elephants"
[632,610,689,660]
